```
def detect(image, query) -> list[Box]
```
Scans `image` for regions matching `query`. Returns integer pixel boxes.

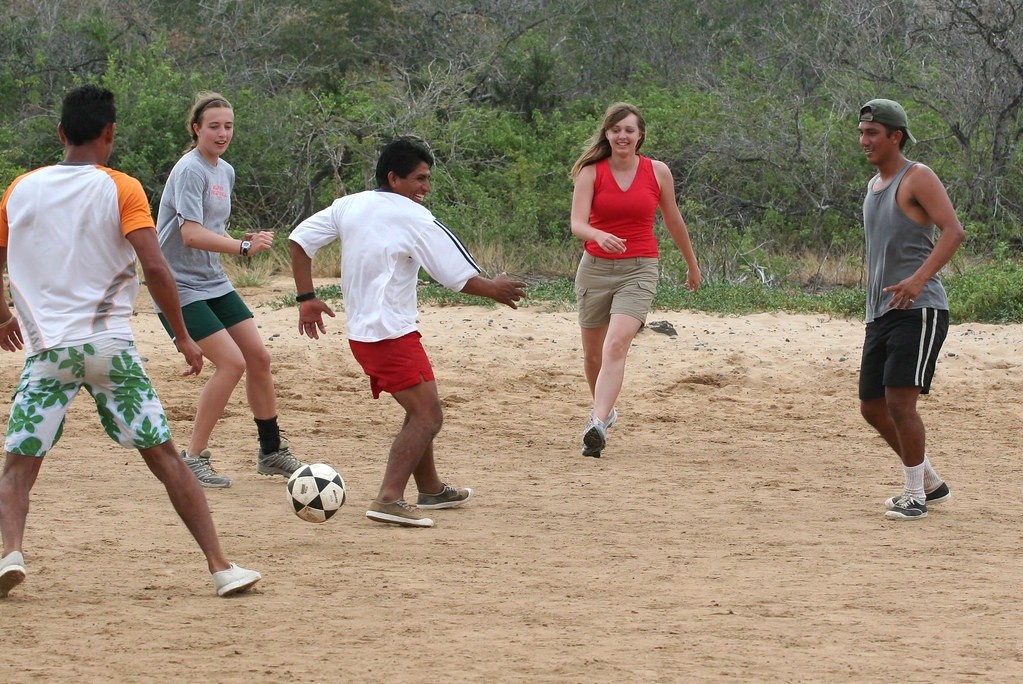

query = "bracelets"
[240,241,243,255]
[0,312,16,329]
[296,292,316,302]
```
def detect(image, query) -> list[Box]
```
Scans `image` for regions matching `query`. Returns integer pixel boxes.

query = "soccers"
[286,463,346,523]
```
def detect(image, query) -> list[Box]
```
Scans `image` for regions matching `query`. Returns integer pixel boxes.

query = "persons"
[151,91,308,488]
[858,99,964,518]
[288,135,527,527]
[567,103,702,458]
[0,86,262,603]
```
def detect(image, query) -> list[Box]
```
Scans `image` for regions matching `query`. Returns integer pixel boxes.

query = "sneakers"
[581,417,606,458]
[256,438,306,479]
[884,480,951,508]
[180,449,231,488]
[0,551,26,597]
[885,495,928,521]
[366,496,434,527]
[212,561,261,597]
[586,406,617,430]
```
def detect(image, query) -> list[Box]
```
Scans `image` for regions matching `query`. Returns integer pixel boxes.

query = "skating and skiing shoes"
[415,482,473,510]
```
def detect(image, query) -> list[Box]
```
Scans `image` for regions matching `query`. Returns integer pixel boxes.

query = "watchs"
[242,240,252,256]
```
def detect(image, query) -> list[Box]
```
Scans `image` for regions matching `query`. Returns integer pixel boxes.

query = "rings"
[908,299,914,304]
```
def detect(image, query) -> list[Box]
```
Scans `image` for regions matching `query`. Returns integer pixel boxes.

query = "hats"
[857,98,917,147]
